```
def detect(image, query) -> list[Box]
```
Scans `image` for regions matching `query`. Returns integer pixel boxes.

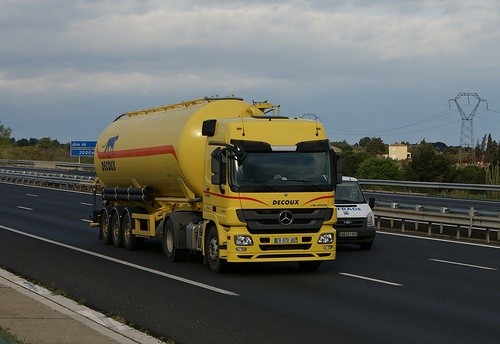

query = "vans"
[332,176,378,251]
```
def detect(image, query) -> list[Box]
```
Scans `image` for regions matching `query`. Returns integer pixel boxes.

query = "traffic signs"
[69,149,94,157]
[70,140,97,148]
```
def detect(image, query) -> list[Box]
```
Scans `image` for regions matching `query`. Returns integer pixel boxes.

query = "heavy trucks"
[89,95,337,273]
